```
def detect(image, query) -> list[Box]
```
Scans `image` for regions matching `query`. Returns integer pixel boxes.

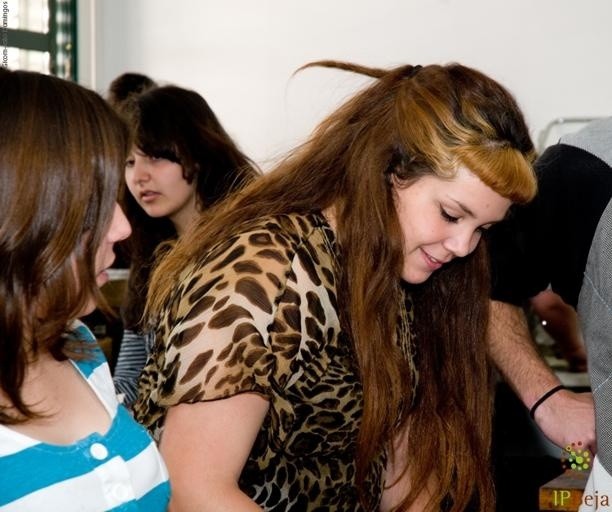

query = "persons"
[478,115,611,512]
[0,62,173,512]
[134,58,539,511]
[101,72,161,377]
[104,82,267,411]
